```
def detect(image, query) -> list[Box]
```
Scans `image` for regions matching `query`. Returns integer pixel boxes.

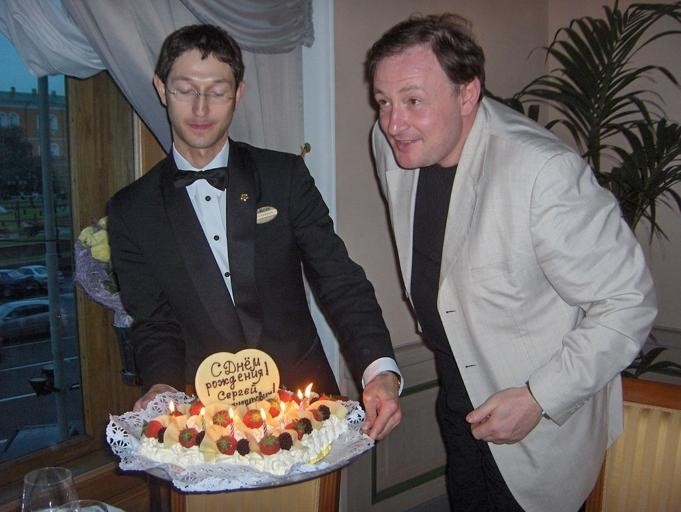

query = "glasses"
[166,88,235,105]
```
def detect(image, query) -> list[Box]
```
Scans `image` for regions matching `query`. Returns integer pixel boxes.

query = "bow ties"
[173,168,228,191]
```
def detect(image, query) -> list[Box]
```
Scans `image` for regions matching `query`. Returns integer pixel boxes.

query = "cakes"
[136,388,349,477]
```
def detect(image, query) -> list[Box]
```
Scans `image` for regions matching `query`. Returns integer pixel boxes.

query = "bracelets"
[384,367,403,390]
[524,379,555,420]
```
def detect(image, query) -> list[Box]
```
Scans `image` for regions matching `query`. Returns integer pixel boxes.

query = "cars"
[0,299,68,343]
[21,264,66,291]
[1,268,41,300]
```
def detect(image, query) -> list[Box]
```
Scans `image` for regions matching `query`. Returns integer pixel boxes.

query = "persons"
[361,11,662,512]
[104,24,406,446]
